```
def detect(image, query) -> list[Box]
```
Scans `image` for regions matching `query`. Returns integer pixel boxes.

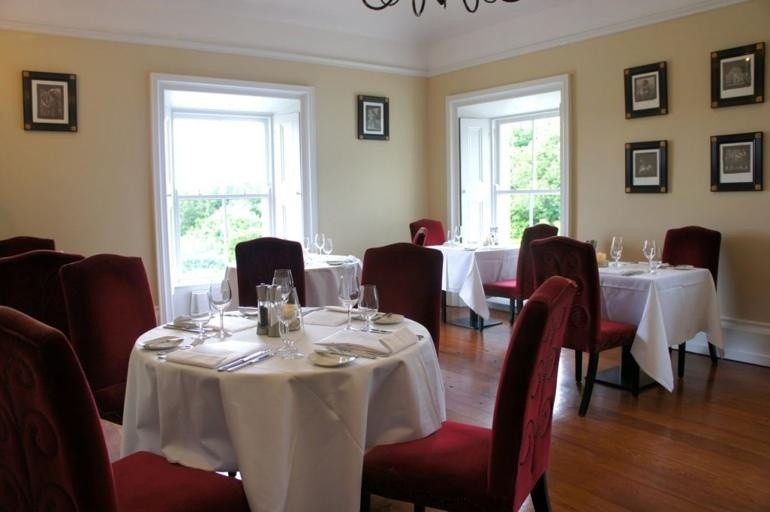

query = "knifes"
[163,324,212,333]
[218,350,274,372]
[370,329,423,341]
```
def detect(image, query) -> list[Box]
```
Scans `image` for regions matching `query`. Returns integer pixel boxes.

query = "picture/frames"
[710,131,764,192]
[624,140,667,193]
[624,60,668,119]
[710,40,766,108]
[21,70,79,132]
[358,94,390,140]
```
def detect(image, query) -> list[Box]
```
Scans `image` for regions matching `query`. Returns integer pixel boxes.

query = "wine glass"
[337,275,360,332]
[188,290,212,340]
[448,230,455,246]
[643,239,656,272]
[209,279,232,338]
[610,248,622,265]
[649,248,662,275]
[278,303,304,359]
[272,278,289,303]
[315,233,325,257]
[275,286,296,352]
[611,236,623,267]
[359,285,379,332]
[455,226,463,242]
[322,239,332,256]
[274,269,294,303]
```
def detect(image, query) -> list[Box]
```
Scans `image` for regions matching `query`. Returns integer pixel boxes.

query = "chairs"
[359,242,444,356]
[0,250,87,342]
[409,217,447,324]
[1,236,56,256]
[235,236,307,306]
[0,303,250,512]
[528,236,640,418]
[413,227,426,245]
[361,275,579,512]
[662,226,722,378]
[58,253,157,425]
[478,223,559,333]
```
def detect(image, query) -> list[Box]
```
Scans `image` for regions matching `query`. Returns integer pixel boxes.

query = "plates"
[307,352,356,367]
[138,335,183,349]
[373,313,403,325]
